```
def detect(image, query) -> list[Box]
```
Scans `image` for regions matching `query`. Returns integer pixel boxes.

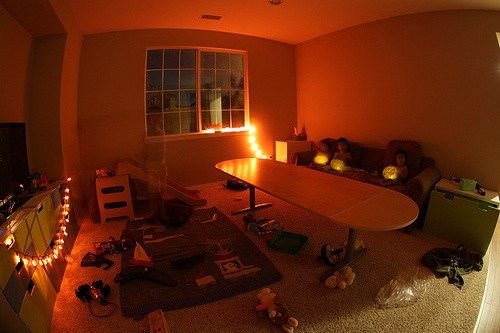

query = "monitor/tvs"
[0,121,33,218]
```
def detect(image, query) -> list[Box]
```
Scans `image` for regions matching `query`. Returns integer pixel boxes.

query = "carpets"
[118,206,283,321]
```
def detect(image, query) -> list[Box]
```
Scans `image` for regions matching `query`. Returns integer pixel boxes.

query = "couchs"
[290,137,441,234]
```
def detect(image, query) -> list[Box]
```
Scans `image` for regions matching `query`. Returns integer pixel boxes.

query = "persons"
[309,139,332,171]
[320,137,353,171]
[376,149,408,187]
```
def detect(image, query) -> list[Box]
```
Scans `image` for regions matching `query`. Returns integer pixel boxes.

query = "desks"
[276,140,311,163]
[215,157,419,282]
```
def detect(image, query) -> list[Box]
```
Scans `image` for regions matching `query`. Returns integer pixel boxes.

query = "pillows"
[383,140,421,182]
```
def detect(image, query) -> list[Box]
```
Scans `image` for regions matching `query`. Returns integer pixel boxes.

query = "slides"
[129,157,208,207]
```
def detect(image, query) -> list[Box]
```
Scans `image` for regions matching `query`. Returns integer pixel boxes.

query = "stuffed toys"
[254,287,298,333]
[320,265,355,289]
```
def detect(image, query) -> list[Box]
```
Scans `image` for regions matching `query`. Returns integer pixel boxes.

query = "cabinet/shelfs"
[423,178,500,256]
[0,184,79,333]
